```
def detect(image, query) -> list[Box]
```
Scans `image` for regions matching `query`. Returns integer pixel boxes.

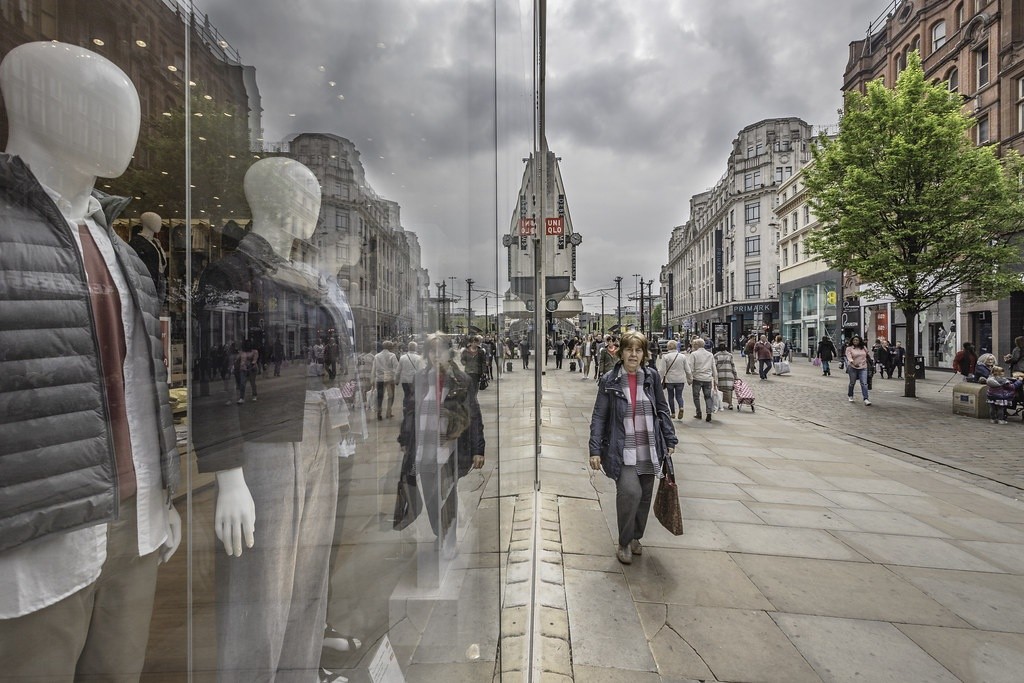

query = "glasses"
[589,467,606,494]
[468,466,486,493]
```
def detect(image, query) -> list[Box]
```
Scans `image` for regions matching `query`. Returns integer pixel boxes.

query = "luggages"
[570,356,576,371]
[734,379,756,413]
[507,355,512,371]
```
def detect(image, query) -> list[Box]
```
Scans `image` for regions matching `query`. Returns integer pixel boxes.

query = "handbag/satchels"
[813,358,821,366]
[654,454,683,536]
[774,361,790,375]
[712,386,724,413]
[480,372,488,390]
[662,376,667,389]
[393,451,422,531]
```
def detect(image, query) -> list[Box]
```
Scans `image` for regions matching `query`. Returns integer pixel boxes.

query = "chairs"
[965,376,1024,423]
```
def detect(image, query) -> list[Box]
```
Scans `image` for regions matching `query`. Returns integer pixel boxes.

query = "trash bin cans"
[904,355,925,379]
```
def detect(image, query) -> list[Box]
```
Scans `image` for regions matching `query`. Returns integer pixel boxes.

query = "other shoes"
[617,541,632,564]
[632,537,643,555]
[849,396,854,402]
[670,414,675,418]
[706,413,711,422]
[998,419,1008,424]
[864,399,871,406]
[760,373,767,379]
[746,371,758,375]
[678,409,684,419]
[695,412,702,418]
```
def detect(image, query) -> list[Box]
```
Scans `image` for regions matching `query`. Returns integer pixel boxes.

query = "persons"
[658,339,693,419]
[589,331,679,564]
[873,338,905,379]
[0,42,183,683]
[688,339,719,421]
[191,157,371,683]
[129,212,167,309]
[937,319,956,353]
[690,335,713,353]
[846,336,872,405]
[553,332,621,380]
[199,331,530,556]
[817,335,837,376]
[739,334,792,380]
[841,342,848,362]
[953,335,1024,424]
[714,343,737,410]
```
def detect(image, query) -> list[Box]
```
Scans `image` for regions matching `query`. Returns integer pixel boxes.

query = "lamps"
[92,19,106,46]
[190,69,199,86]
[135,28,146,47]
[768,223,781,229]
[204,82,214,100]
[167,56,179,72]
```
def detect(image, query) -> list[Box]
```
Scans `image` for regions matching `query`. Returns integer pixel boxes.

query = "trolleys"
[734,378,756,413]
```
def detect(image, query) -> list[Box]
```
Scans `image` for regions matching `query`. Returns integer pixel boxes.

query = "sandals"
[322,622,356,652]
[318,667,349,683]
